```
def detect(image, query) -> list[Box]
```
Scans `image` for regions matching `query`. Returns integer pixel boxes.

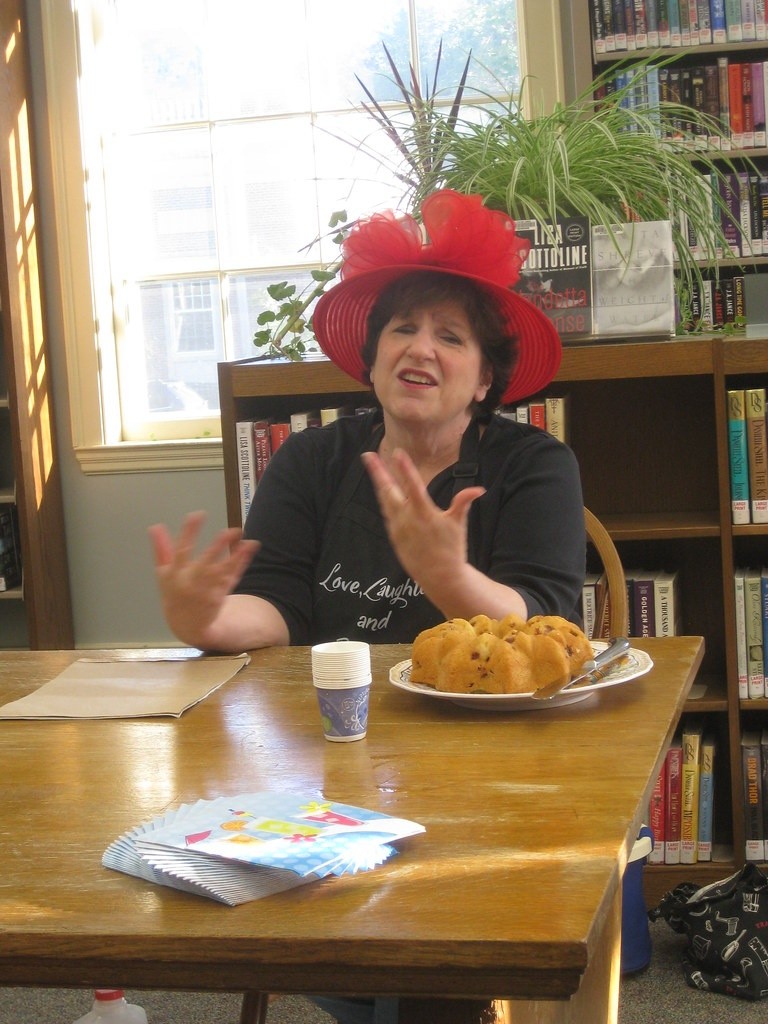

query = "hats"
[311,188,562,404]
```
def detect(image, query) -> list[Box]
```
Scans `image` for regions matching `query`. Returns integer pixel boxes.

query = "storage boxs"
[618,832,660,979]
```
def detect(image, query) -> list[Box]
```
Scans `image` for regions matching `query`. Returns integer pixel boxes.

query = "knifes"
[530,637,630,701]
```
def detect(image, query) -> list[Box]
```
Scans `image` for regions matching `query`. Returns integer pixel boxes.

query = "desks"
[0,632,705,1024]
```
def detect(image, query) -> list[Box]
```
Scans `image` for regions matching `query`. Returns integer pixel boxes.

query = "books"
[236,405,379,534]
[674,271,746,336]
[590,221,675,337]
[618,169,768,261]
[643,724,719,864]
[595,56,768,154]
[742,730,768,861]
[503,216,594,337]
[577,569,684,638]
[497,395,572,449]
[726,386,768,524]
[0,653,250,720]
[589,0,768,52]
[735,566,768,699]
[0,510,22,591]
[102,791,426,906]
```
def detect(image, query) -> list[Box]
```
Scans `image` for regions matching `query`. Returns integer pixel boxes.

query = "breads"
[408,614,595,695]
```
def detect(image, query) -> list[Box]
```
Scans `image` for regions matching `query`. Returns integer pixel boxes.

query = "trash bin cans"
[619,823,656,978]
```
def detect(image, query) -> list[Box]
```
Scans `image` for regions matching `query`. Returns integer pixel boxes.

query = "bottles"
[73,990,148,1024]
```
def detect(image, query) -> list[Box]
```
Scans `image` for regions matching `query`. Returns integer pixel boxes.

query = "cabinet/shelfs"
[562,0,768,327]
[212,327,768,913]
[0,0,72,652]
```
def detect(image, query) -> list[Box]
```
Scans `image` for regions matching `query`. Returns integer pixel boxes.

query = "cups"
[311,641,373,742]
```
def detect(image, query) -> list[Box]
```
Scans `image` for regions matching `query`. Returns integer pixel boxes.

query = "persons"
[150,192,587,1024]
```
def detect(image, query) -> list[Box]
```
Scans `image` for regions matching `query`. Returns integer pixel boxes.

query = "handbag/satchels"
[646,861,768,1000]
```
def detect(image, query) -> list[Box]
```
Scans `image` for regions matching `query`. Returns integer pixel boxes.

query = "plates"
[388,641,654,711]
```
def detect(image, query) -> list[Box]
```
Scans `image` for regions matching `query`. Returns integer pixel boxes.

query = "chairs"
[242,506,629,1024]
